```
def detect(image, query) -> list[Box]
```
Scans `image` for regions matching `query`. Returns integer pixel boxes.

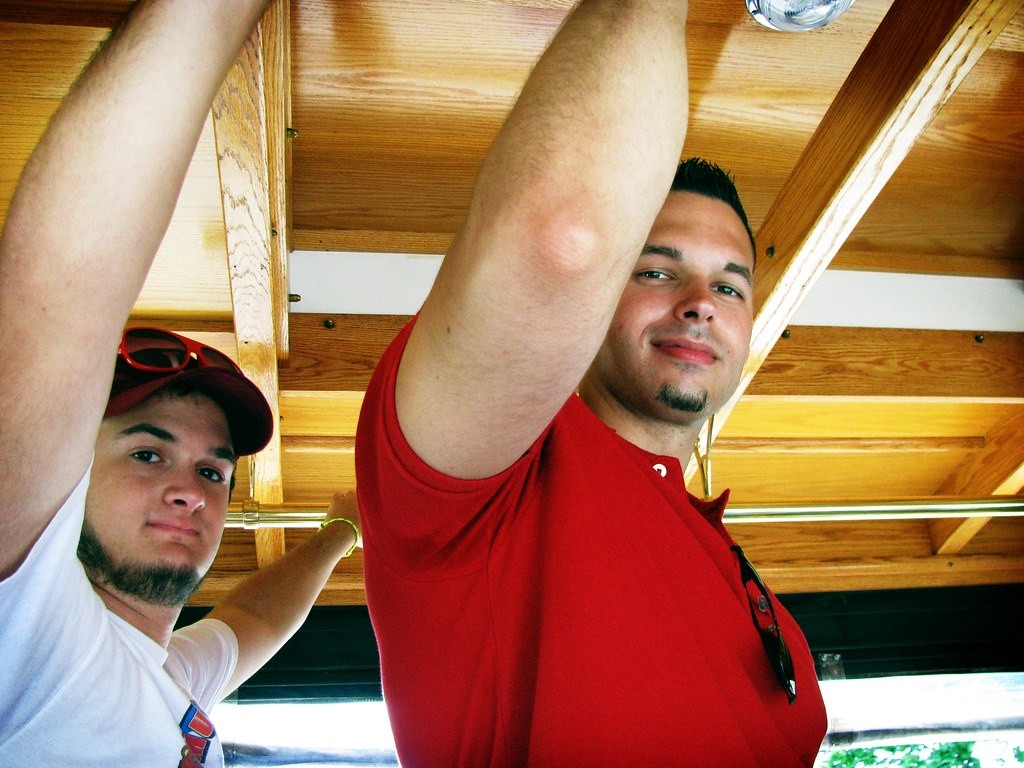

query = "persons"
[358,0,827,767]
[0,0,365,768]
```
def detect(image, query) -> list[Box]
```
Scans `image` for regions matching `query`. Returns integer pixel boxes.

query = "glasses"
[731,543,797,707]
[117,326,245,378]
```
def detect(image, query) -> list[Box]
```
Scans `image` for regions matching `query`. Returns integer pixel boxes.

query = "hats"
[104,355,273,461]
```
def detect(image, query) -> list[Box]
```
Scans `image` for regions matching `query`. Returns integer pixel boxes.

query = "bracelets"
[317,519,359,557]
